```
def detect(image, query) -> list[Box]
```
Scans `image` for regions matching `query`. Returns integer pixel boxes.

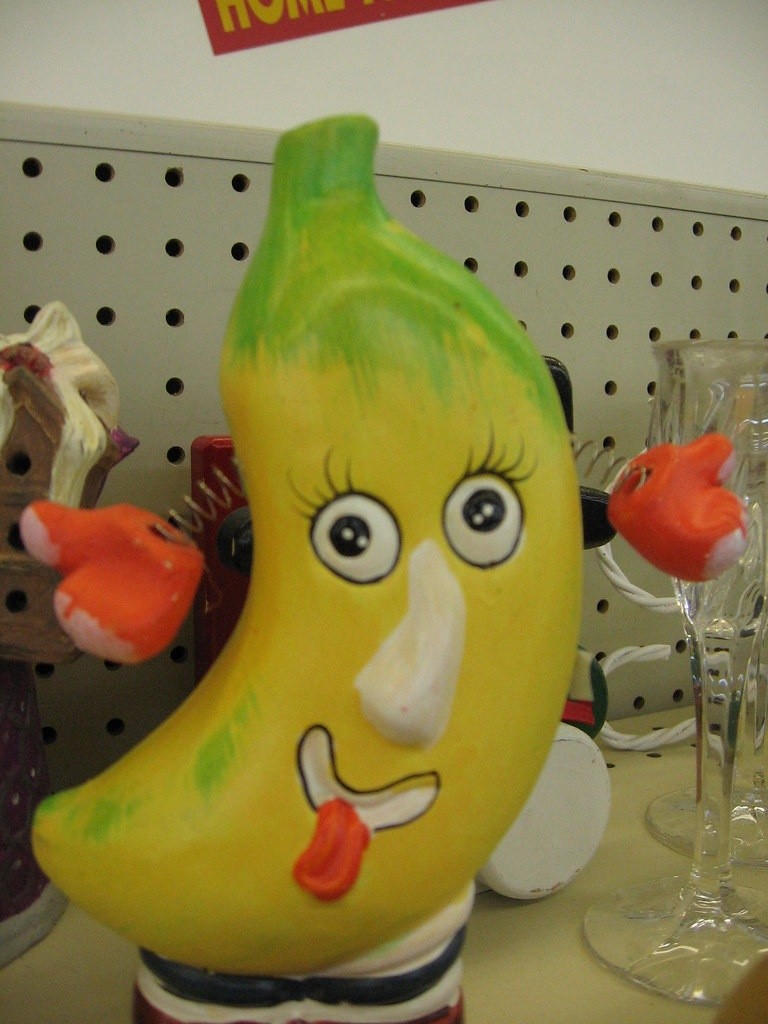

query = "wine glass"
[579,341,767,1004]
